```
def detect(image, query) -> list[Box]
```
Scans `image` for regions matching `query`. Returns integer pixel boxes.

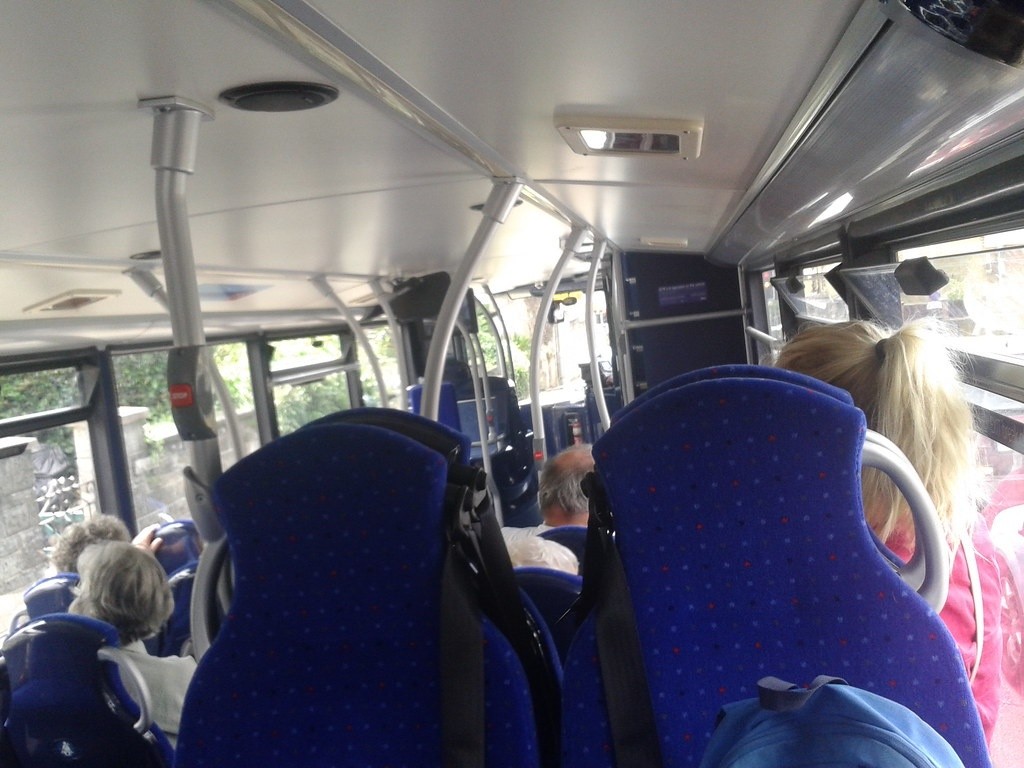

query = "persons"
[757,322,998,749]
[493,536,578,577]
[496,443,595,547]
[53,516,161,593]
[58,544,197,758]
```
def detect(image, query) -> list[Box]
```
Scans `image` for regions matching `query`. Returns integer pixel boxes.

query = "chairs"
[3,375,993,766]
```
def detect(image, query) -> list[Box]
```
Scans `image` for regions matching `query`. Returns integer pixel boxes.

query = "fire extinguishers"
[569,418,584,448]
